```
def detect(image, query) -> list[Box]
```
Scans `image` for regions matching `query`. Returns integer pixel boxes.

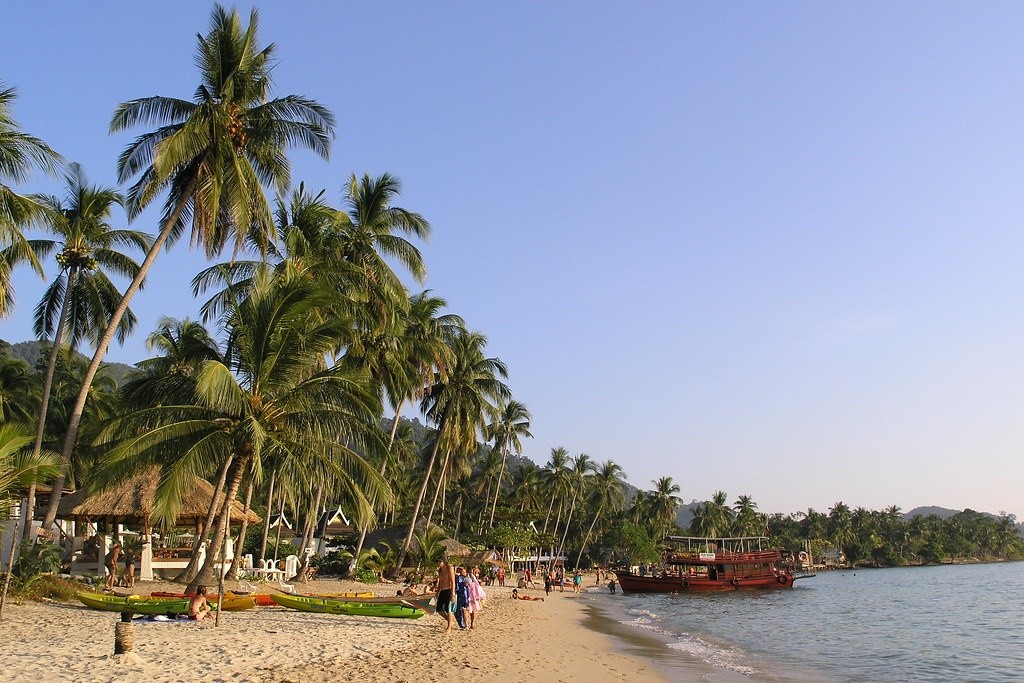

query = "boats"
[610,532,817,595]
[248,593,377,606]
[263,582,438,614]
[75,590,218,617]
[151,590,257,611]
[270,593,425,620]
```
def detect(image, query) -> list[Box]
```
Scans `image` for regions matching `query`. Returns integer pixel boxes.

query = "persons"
[512,567,618,601]
[639,563,694,579]
[434,556,455,634]
[454,568,485,631]
[123,547,141,587]
[473,564,506,586]
[104,545,121,587]
[403,581,437,596]
[189,585,214,620]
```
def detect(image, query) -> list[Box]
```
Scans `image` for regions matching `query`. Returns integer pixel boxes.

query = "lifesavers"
[732,579,739,586]
[797,551,808,562]
[779,574,788,585]
[681,580,690,588]
